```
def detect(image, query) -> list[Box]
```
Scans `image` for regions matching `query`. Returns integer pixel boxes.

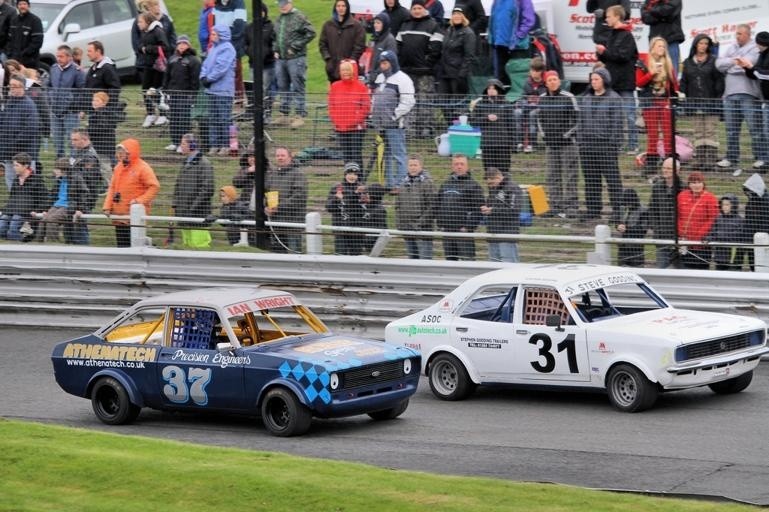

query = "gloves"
[202,77,210,89]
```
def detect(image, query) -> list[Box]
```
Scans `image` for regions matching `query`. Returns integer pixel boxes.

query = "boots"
[691,139,719,170]
[639,153,679,175]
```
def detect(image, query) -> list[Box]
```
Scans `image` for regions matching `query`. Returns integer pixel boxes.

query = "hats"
[543,71,559,83]
[219,186,237,200]
[343,163,361,175]
[453,4,466,14]
[412,0,424,7]
[176,34,192,47]
[277,1,293,8]
[755,32,769,47]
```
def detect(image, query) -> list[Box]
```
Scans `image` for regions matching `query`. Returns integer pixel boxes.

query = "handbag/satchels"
[152,57,169,73]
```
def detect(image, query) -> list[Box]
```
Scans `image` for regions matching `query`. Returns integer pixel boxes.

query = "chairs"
[228,80,274,147]
[158,90,210,138]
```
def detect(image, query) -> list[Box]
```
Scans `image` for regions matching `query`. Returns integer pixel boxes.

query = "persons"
[1,1,768,270]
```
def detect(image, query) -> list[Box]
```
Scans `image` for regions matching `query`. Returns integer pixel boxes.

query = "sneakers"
[384,187,400,195]
[142,115,167,128]
[208,148,229,156]
[625,144,639,157]
[539,208,621,224]
[718,159,732,167]
[165,144,184,153]
[98,185,108,196]
[752,160,768,168]
[241,112,305,129]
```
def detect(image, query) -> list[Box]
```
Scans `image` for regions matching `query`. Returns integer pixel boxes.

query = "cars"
[0,0,173,91]
[383,261,768,411]
[49,286,421,437]
